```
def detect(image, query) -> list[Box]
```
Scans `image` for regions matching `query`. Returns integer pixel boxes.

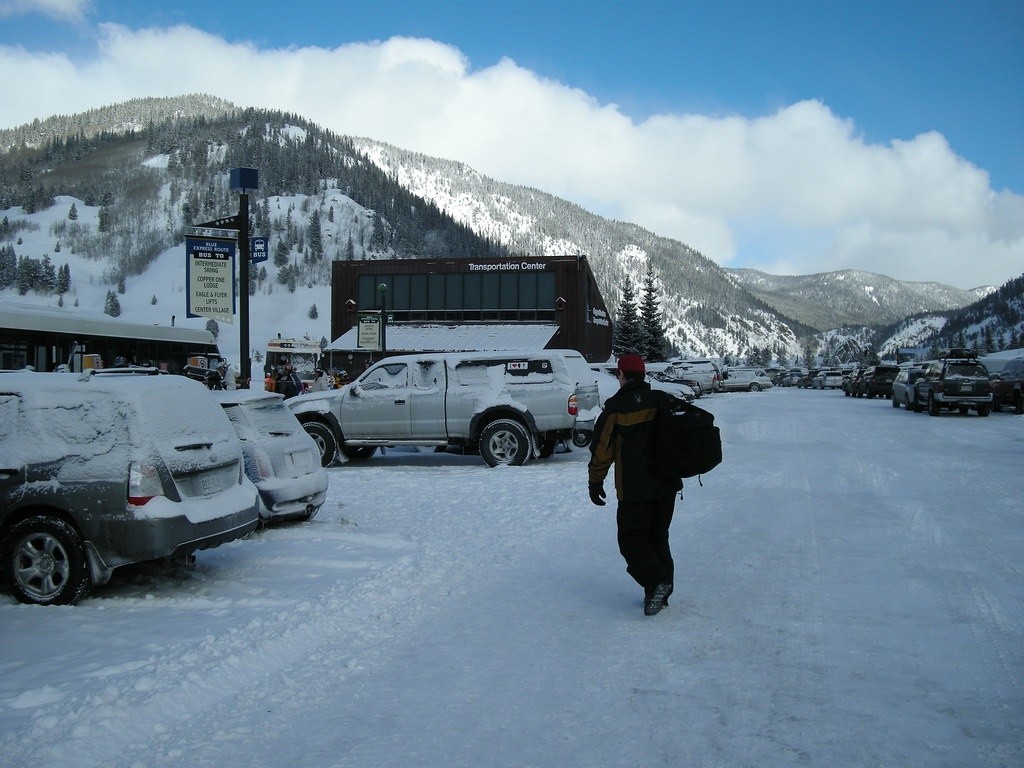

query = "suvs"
[283,349,600,470]
[891,362,940,412]
[857,364,901,399]
[0,363,260,606]
[989,357,1024,415]
[912,346,996,417]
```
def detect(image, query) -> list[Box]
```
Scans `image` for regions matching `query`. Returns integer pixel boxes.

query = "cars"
[587,354,867,414]
[211,388,328,533]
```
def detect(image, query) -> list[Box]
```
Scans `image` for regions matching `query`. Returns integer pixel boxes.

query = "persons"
[587,352,684,616]
[265,363,364,401]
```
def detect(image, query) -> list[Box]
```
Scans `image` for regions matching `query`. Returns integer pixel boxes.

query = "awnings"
[322,325,559,352]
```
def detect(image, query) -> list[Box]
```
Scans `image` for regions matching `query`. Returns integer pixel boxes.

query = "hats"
[617,354,646,373]
[364,359,373,363]
[266,373,271,377]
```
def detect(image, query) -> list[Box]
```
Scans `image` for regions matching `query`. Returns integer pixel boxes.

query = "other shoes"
[643,571,672,615]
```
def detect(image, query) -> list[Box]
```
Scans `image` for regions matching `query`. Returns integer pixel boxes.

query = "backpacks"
[651,389,722,477]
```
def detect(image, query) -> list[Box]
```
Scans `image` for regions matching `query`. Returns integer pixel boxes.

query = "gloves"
[587,481,607,507]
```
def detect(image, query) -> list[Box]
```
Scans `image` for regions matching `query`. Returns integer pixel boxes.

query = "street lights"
[229,165,261,385]
